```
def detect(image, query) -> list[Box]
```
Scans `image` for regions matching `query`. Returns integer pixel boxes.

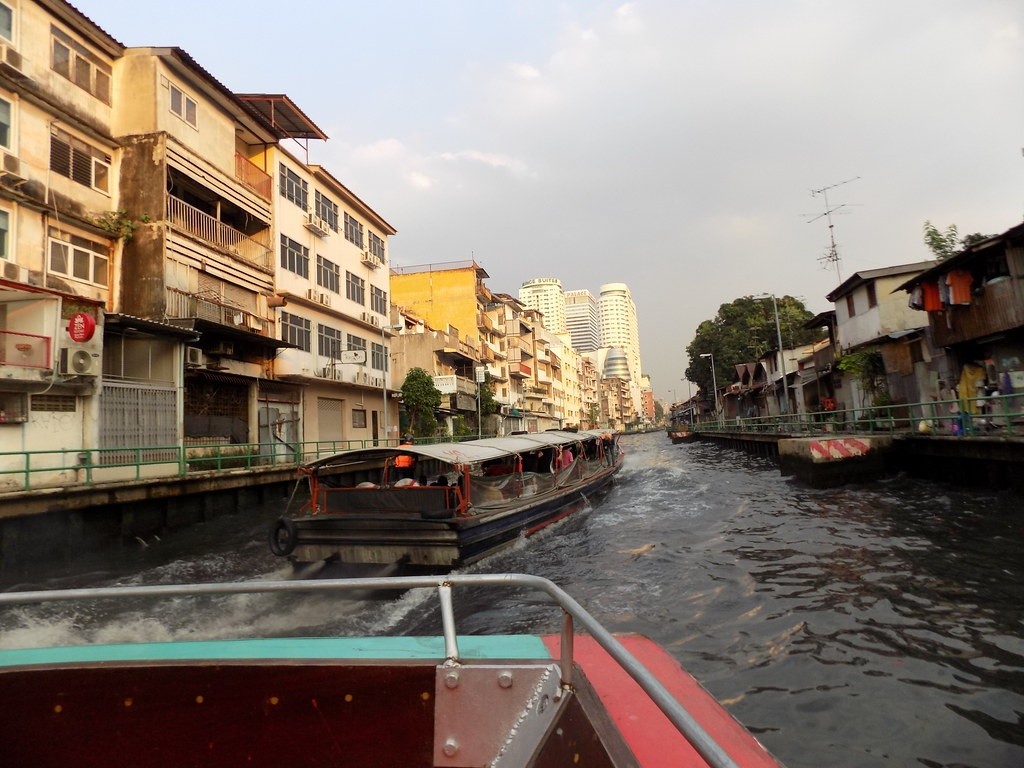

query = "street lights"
[685,377,694,430]
[763,292,793,433]
[699,353,719,421]
[668,389,677,405]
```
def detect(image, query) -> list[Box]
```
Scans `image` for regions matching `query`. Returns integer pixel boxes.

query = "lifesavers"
[394,477,420,486]
[358,482,375,488]
[601,433,612,440]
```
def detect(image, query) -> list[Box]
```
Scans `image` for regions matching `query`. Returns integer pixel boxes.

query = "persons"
[418,474,464,508]
[481,445,574,484]
[747,400,762,431]
[937,380,964,435]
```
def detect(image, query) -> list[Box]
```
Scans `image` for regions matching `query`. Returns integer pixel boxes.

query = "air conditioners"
[0,149,20,176]
[58,347,100,377]
[319,294,330,306]
[307,213,320,227]
[306,288,319,302]
[364,251,374,262]
[370,315,379,326]
[373,256,381,267]
[320,220,329,233]
[184,346,202,366]
[362,311,370,322]
[0,44,23,71]
[0,258,20,282]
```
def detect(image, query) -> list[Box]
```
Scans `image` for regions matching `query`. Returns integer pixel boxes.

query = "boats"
[277,427,625,570]
